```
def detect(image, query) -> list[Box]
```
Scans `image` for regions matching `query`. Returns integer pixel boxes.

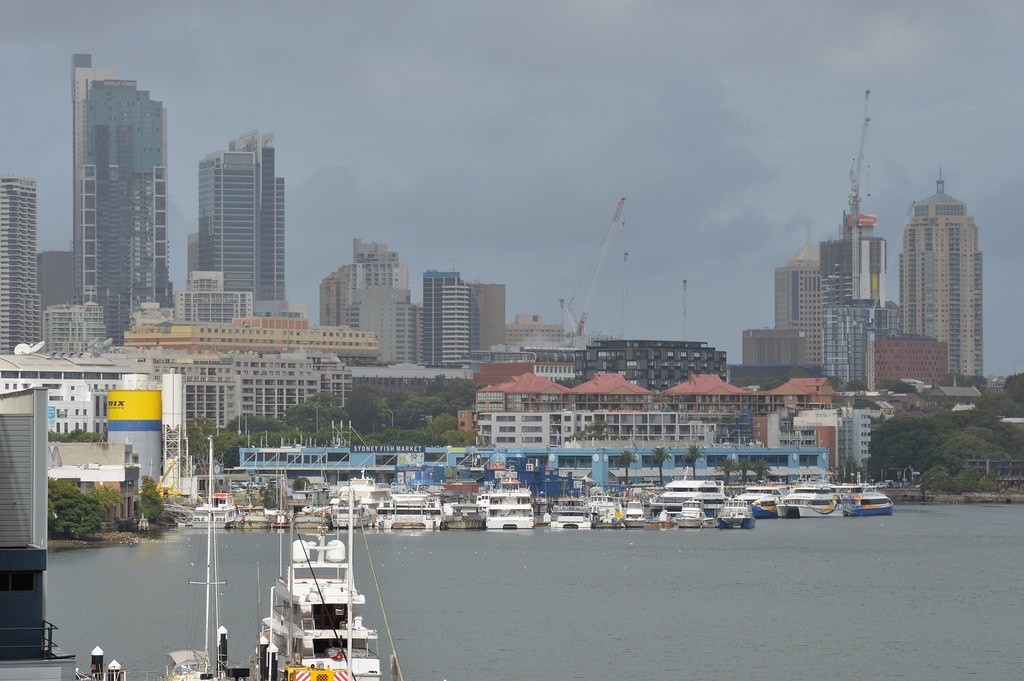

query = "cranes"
[559,195,687,339]
[841,86,874,216]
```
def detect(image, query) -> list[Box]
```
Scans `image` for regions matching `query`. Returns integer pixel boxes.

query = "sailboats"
[176,416,895,532]
[158,430,226,681]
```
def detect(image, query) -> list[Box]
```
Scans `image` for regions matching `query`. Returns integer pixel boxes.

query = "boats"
[254,482,404,681]
